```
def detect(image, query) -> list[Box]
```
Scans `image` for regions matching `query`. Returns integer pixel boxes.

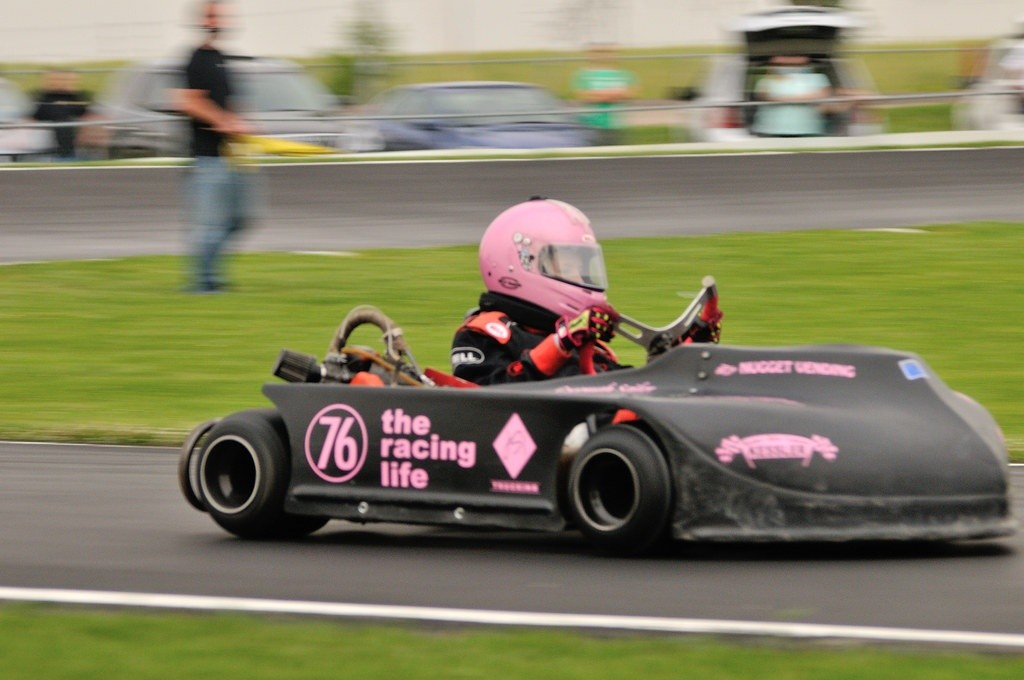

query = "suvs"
[103,50,388,162]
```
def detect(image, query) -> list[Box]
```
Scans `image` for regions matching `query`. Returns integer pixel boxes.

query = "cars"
[356,79,610,153]
[640,52,888,143]
[0,73,60,167]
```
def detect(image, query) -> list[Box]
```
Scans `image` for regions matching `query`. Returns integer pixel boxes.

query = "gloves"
[552,304,620,359]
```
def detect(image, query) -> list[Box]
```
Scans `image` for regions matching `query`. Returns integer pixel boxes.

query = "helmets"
[479,197,608,324]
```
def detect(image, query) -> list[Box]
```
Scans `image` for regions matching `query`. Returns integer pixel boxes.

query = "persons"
[162,0,261,296]
[449,196,723,384]
[573,40,645,145]
[749,55,866,138]
[35,71,93,160]
